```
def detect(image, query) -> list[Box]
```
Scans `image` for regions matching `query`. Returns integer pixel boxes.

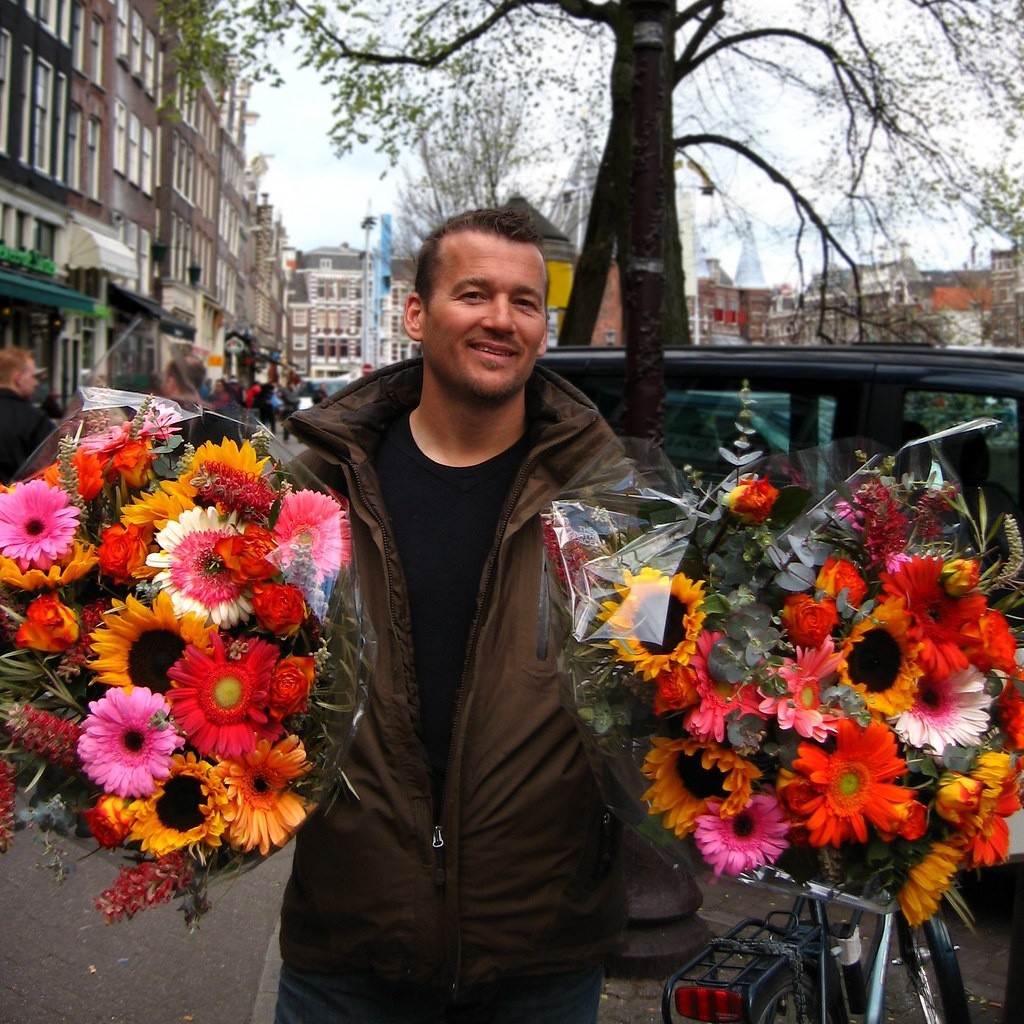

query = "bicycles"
[662,835,969,1024]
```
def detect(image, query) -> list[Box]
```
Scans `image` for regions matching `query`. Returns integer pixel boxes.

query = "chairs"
[901,420,955,547]
[940,424,1024,563]
[718,431,771,477]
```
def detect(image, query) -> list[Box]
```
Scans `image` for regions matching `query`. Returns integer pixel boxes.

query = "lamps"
[189,261,202,285]
[151,238,169,262]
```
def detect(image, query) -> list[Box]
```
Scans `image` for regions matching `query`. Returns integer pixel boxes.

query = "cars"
[536,345,1024,647]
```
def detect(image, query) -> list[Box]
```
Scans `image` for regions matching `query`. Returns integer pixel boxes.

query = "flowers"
[539,378,1024,934]
[0,384,351,935]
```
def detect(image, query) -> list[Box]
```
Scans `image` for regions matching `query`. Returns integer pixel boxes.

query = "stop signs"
[362,364,373,376]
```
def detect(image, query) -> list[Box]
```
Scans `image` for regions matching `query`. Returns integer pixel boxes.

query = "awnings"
[66,224,139,281]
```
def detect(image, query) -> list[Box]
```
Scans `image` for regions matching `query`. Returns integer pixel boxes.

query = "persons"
[258,206,664,1024]
[0,345,58,485]
[204,377,329,444]
[158,352,214,410]
